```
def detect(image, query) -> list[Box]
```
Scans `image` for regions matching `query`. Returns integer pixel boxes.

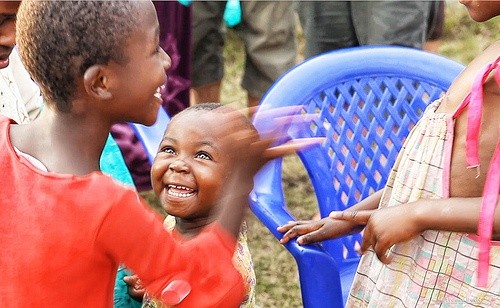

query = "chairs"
[247,43,465,308]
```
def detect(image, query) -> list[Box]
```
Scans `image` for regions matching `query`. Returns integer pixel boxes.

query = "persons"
[110,0,294,185]
[124,104,259,308]
[276,0,500,308]
[0,0,326,308]
[298,0,428,260]
[0,0,47,124]
